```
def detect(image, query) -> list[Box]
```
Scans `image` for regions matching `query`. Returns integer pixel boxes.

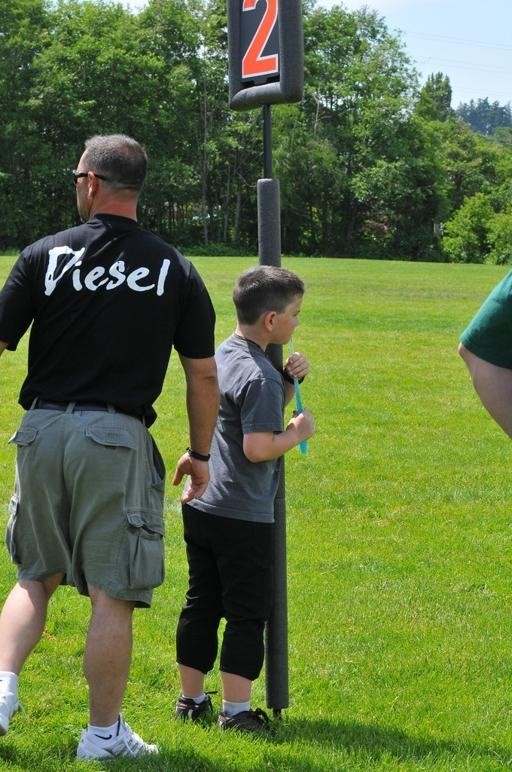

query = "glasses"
[73,170,105,183]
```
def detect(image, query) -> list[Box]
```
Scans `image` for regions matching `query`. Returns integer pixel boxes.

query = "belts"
[35,398,134,416]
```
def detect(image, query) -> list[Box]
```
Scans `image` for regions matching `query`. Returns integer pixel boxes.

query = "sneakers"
[77,714,159,758]
[0,692,29,736]
[176,691,276,737]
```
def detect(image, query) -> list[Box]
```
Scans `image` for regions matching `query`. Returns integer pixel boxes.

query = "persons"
[175,265,315,738]
[458,268,512,440]
[0,133,220,761]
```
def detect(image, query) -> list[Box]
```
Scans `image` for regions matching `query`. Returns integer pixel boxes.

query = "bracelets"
[186,447,211,462]
[282,369,303,386]
